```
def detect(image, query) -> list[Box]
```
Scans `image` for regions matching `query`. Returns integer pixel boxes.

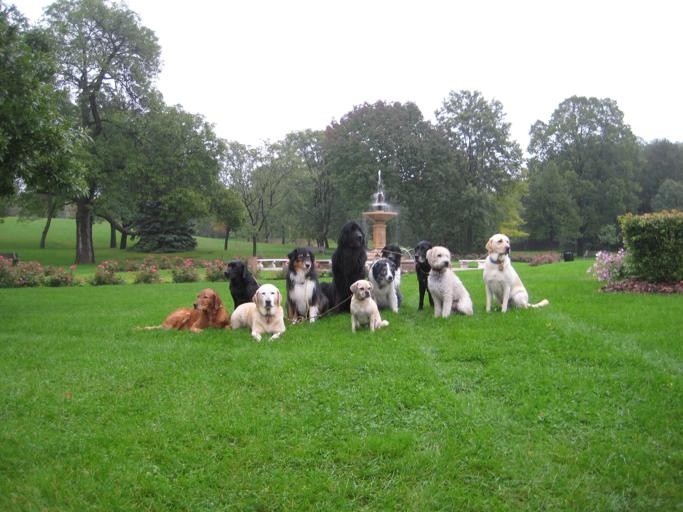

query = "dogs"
[415,240,473,319]
[224,261,286,342]
[483,233,549,313]
[136,288,232,334]
[287,220,401,334]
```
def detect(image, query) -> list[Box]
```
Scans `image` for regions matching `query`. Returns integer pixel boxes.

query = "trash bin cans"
[564,250,573,261]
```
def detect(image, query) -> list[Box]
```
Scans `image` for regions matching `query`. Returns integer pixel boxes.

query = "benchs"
[459,259,487,270]
[315,260,330,269]
[256,259,290,271]
